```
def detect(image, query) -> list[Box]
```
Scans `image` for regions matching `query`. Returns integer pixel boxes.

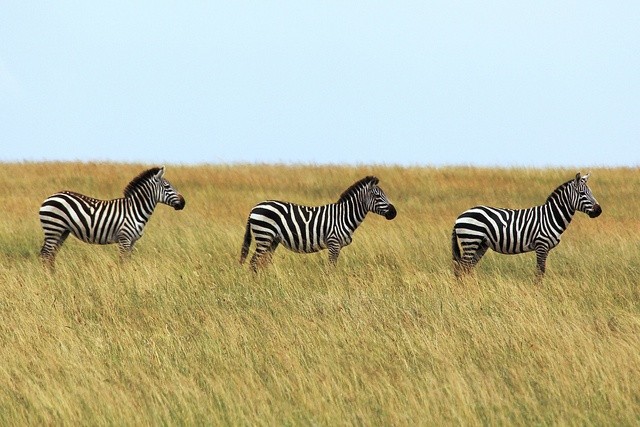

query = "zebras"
[240,176,397,277]
[452,173,602,285]
[37,167,185,272]
